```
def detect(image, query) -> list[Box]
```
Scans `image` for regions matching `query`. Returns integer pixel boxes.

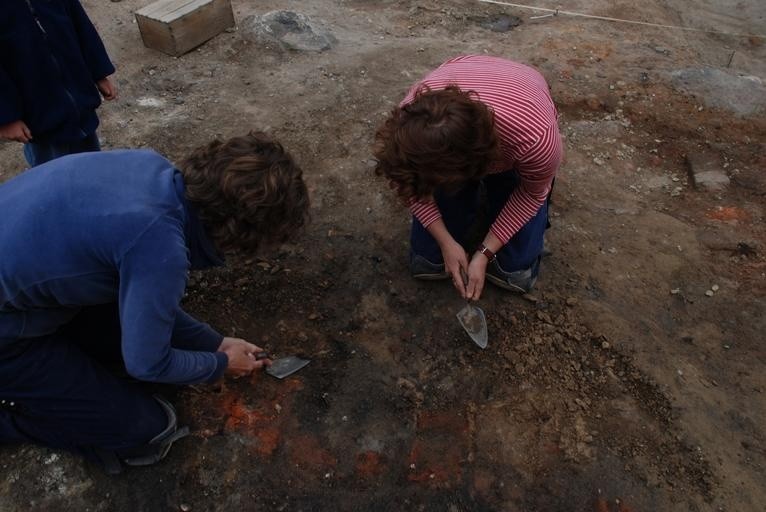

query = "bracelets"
[477,243,496,265]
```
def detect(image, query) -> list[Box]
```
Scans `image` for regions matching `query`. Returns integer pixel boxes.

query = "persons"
[0,126,313,472]
[365,51,564,305]
[0,1,120,167]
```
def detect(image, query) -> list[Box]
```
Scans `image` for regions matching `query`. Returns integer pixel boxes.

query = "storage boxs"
[133,2,238,58]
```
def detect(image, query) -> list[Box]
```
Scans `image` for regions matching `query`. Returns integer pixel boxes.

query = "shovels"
[254,350,311,380]
[456,267,488,350]
